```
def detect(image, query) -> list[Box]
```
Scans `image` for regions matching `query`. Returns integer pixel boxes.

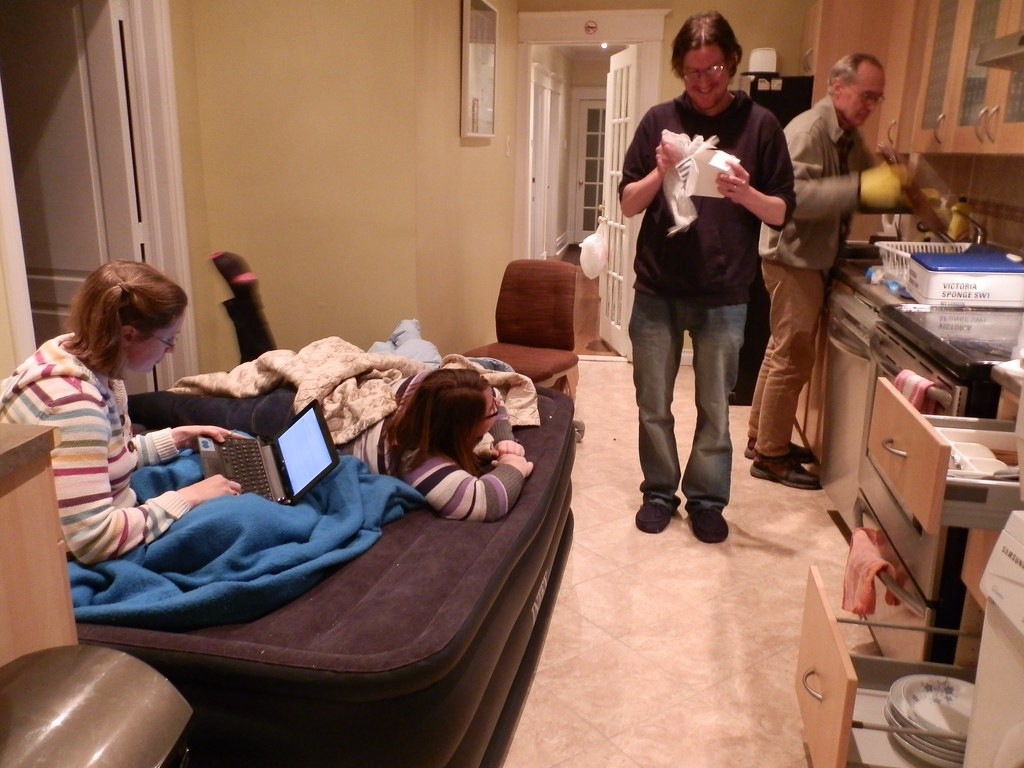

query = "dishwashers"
[818,278,879,534]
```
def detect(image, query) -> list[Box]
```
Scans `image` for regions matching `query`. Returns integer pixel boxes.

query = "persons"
[0,260,251,566]
[617,8,796,543]
[123,253,534,521]
[744,53,940,489]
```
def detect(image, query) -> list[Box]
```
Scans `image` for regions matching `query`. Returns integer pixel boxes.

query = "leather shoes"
[746,437,813,463]
[750,454,824,490]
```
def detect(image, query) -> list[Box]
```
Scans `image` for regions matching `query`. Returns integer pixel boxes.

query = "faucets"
[950,205,989,253]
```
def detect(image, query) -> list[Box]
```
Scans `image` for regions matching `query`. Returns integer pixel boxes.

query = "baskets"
[874,241,1021,284]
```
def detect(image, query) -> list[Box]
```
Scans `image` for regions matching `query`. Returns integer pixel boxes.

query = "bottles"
[947,197,969,240]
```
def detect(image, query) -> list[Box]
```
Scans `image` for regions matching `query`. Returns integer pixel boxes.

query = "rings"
[232,490,237,496]
[733,184,737,192]
[227,431,232,437]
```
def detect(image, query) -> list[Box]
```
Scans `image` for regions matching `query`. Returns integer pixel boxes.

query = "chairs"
[460,260,587,442]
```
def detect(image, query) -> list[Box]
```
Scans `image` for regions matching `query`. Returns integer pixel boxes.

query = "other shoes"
[636,504,671,533]
[685,499,728,544]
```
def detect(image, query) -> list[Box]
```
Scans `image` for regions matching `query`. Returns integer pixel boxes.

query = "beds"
[77,392,577,768]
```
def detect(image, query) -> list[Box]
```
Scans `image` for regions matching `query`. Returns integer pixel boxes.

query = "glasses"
[475,387,499,421]
[848,87,886,107]
[133,328,178,351]
[679,55,727,82]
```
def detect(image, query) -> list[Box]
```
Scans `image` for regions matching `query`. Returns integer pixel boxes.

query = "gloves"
[858,159,911,208]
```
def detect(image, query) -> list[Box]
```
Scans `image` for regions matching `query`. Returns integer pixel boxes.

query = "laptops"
[197,400,340,506]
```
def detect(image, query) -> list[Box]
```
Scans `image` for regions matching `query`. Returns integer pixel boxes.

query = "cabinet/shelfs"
[799,0,1024,156]
[787,280,858,464]
[791,368,1024,768]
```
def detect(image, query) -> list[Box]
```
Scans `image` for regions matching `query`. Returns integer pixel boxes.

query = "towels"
[892,369,938,414]
[62,333,542,616]
[838,527,898,621]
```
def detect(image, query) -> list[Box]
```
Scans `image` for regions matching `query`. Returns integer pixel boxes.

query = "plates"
[883,673,975,767]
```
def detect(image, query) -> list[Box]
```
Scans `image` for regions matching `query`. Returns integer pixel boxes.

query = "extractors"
[975,29,1024,73]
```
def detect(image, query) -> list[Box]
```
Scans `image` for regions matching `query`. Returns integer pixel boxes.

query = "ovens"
[854,321,1000,664]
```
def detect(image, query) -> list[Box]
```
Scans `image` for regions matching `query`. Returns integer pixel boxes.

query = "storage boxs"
[906,252,1024,308]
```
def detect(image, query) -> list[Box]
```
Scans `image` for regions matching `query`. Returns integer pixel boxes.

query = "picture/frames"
[460,0,499,138]
